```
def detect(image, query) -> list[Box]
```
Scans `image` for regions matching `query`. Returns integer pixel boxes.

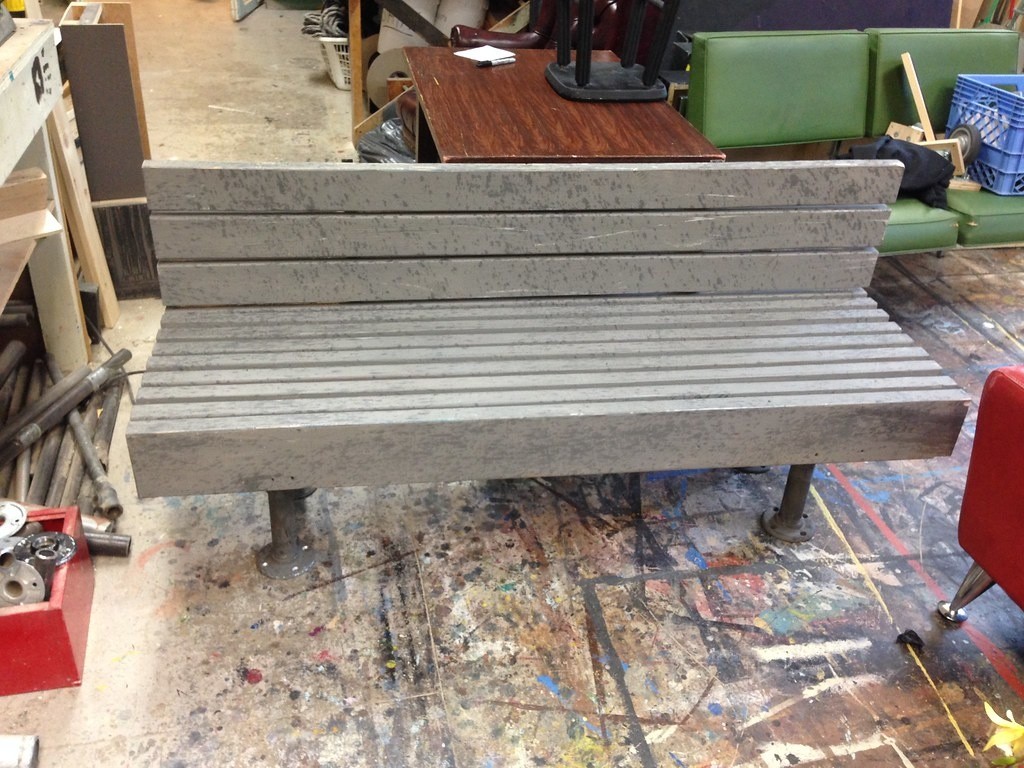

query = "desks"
[400,46,728,164]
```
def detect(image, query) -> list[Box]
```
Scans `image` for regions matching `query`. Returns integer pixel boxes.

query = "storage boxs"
[943,75,1024,196]
[299,30,351,91]
[1,506,95,699]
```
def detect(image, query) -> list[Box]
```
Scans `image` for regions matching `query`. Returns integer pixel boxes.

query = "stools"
[545,0,680,100]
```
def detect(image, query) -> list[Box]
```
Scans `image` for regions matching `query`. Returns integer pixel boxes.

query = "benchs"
[126,158,969,581]
[687,27,1024,257]
